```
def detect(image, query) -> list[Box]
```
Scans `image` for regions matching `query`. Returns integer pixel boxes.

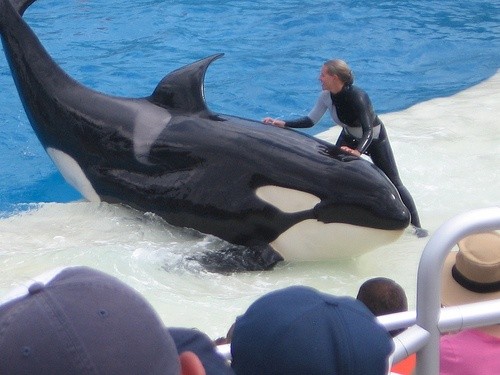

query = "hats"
[166,326,234,375]
[230,285,395,375]
[439,231,500,308]
[0,265,181,375]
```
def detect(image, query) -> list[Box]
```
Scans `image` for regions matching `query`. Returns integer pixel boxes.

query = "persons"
[262,58,429,239]
[357,277,417,375]
[231,285,396,375]
[438,233,500,375]
[0,266,206,375]
